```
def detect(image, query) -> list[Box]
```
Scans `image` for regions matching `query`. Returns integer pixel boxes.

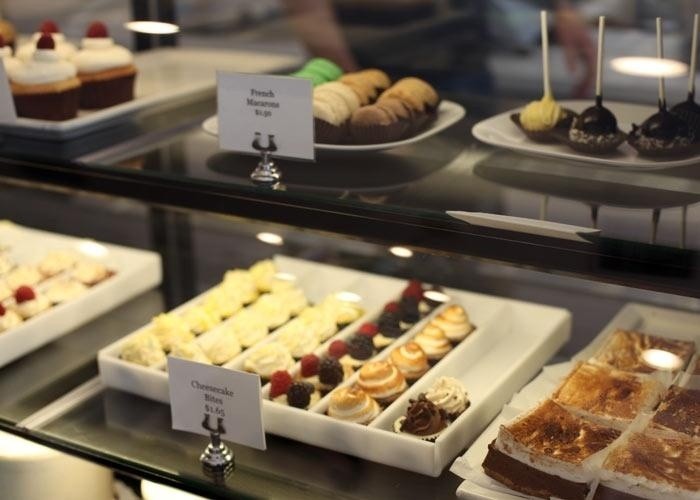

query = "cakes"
[0,19,136,120]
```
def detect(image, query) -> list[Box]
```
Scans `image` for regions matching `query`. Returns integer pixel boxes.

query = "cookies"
[291,57,440,144]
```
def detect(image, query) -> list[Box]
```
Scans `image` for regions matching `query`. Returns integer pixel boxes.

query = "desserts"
[0,221,473,444]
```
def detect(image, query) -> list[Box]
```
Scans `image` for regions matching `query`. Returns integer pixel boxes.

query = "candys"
[519,8,700,153]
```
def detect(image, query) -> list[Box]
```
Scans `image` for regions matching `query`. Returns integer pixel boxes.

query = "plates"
[469,98,700,174]
[92,251,575,479]
[0,215,166,371]
[453,298,700,500]
[2,46,307,141]
[199,98,469,155]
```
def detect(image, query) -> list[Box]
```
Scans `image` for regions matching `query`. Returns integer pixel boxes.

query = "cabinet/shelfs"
[0,0,700,500]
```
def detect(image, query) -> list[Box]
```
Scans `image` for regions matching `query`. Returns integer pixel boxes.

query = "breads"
[479,328,700,500]
